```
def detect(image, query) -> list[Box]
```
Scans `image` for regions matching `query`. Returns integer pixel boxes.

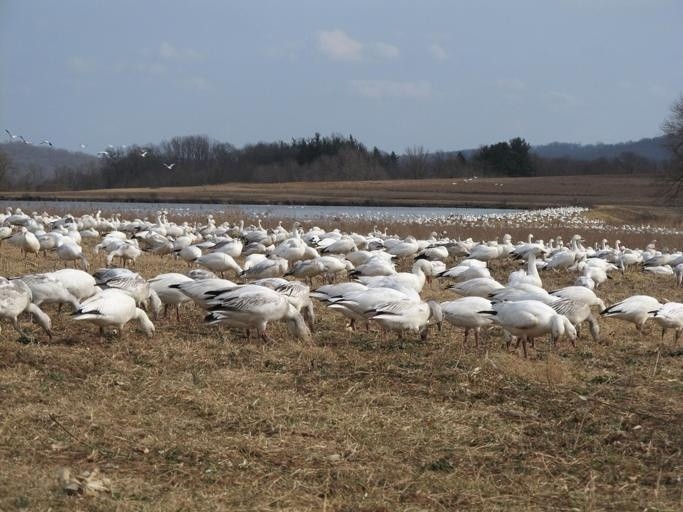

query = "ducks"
[0,206,683,359]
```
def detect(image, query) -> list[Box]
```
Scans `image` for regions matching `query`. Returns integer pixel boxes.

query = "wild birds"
[19,135,32,145]
[97,151,108,158]
[161,163,175,170]
[40,140,52,146]
[139,152,148,157]
[80,143,88,148]
[4,129,18,144]
